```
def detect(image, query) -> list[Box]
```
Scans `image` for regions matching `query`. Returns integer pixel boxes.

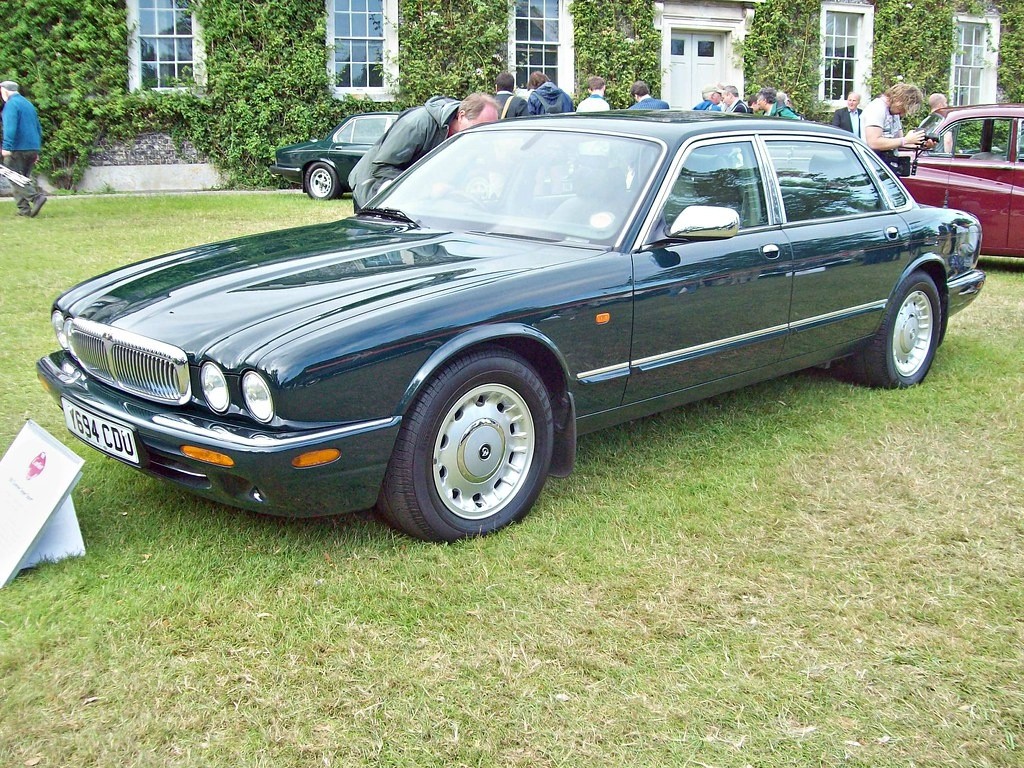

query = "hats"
[702,84,725,94]
[0,81,19,91]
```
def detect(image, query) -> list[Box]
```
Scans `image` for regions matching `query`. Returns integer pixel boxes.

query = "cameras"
[915,128,940,146]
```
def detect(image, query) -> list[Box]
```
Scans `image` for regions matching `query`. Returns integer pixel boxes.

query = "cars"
[36,119,986,541]
[276,111,403,200]
[897,102,1024,267]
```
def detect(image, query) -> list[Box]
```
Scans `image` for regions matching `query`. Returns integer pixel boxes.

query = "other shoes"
[15,208,31,216]
[30,193,47,218]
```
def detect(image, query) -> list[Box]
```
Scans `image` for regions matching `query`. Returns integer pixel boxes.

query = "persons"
[527,70,574,115]
[834,93,863,138]
[0,81,47,217]
[919,93,953,154]
[494,74,529,119]
[859,84,939,165]
[347,94,498,214]
[627,82,669,158]
[757,87,796,119]
[690,84,748,170]
[747,95,759,113]
[576,77,609,157]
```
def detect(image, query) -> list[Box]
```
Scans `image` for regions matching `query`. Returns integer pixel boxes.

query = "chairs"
[615,151,861,228]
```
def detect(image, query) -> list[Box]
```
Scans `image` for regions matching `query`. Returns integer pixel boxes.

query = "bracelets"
[902,137,905,145]
